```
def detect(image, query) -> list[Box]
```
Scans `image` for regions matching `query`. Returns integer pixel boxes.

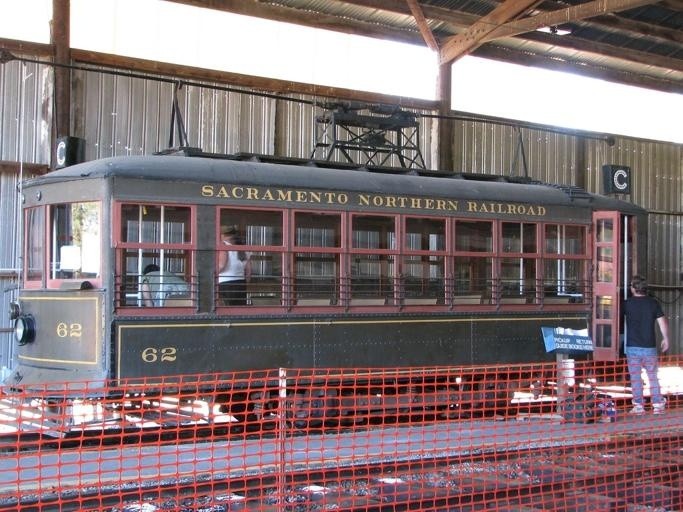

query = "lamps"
[534,14,574,38]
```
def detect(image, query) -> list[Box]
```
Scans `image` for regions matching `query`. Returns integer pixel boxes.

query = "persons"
[142,262,192,307]
[218,223,253,306]
[621,276,669,417]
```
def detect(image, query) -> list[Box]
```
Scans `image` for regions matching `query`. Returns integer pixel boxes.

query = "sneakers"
[628,407,645,416]
[652,403,665,415]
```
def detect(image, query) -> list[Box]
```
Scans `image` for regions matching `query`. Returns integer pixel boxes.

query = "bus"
[0,97,650,436]
